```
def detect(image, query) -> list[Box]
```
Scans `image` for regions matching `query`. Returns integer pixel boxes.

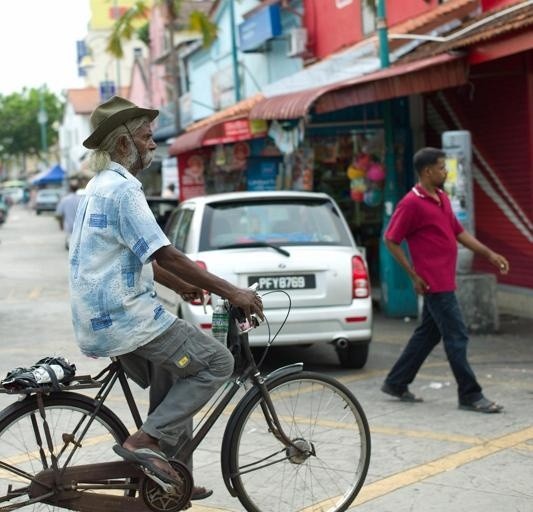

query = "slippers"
[190,486,215,500]
[112,442,186,487]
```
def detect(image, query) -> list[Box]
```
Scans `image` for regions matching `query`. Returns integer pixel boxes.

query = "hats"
[82,95,159,150]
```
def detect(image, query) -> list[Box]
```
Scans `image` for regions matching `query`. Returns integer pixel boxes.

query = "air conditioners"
[284,27,309,58]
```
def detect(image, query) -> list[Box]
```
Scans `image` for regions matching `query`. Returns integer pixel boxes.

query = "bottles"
[210,298,229,347]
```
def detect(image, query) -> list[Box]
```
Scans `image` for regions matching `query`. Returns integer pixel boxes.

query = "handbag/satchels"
[1,355,92,391]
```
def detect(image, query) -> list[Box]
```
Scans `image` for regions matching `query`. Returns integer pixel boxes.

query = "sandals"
[458,395,504,414]
[380,381,425,403]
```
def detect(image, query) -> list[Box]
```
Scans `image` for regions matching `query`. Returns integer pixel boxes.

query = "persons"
[66,93,263,488]
[385,148,510,413]
[54,179,80,236]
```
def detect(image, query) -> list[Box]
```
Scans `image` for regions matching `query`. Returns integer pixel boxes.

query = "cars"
[152,189,371,369]
[34,188,59,215]
[0,182,29,206]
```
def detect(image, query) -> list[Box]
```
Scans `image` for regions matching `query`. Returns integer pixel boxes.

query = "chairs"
[210,218,232,241]
[268,219,290,234]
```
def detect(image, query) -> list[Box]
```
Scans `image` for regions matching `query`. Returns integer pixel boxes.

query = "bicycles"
[0,289,370,508]
